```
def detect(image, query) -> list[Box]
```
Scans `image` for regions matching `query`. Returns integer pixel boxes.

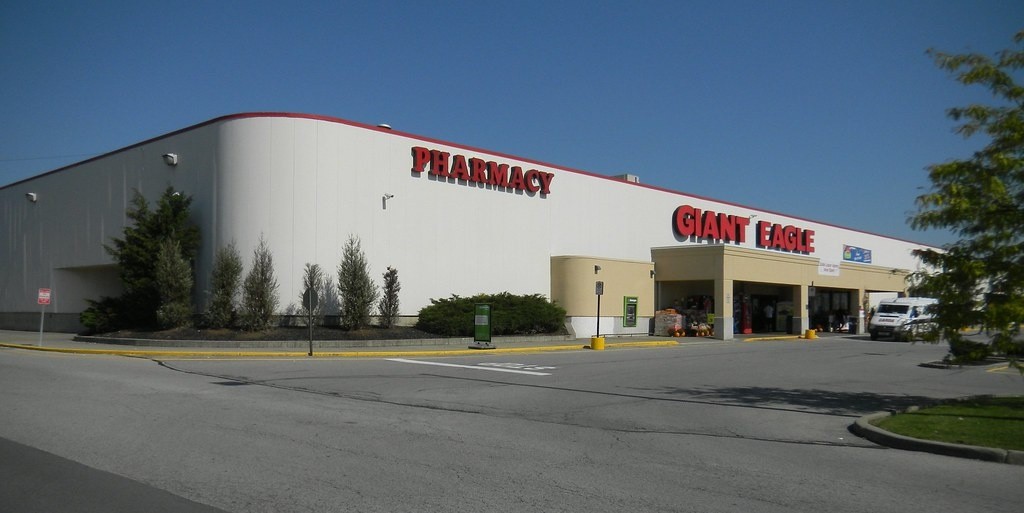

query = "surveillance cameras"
[385,193,394,198]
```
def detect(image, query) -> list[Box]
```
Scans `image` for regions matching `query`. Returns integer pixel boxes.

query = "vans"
[868,298,941,342]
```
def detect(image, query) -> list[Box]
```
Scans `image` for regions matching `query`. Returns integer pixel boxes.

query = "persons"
[833,307,847,332]
[763,304,774,331]
[870,308,875,319]
